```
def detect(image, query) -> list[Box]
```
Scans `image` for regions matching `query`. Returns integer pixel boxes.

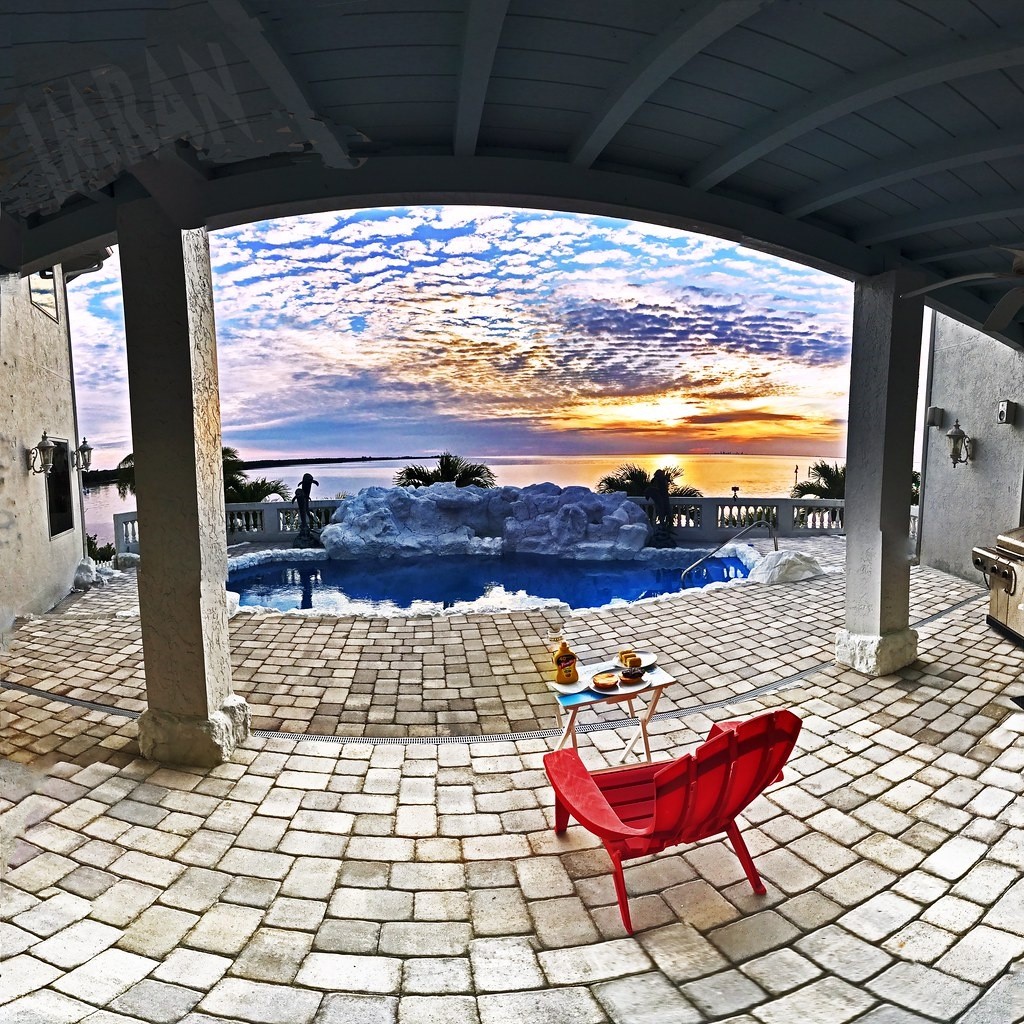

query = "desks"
[551,660,678,764]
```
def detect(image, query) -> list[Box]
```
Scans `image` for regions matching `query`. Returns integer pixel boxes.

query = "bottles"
[552,642,579,683]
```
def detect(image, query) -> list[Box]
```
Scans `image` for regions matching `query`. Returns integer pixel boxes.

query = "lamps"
[71,437,94,473]
[25,431,57,478]
[945,419,973,468]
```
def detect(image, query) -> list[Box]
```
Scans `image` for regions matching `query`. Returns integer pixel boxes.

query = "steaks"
[622,667,645,678]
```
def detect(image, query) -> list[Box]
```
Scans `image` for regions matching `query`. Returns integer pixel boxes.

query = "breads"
[619,674,639,683]
[619,650,641,668]
[594,673,618,689]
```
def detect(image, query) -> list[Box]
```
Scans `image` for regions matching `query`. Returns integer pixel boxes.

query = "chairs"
[543,708,804,935]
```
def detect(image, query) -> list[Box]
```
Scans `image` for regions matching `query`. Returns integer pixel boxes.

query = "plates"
[588,668,653,696]
[613,650,658,668]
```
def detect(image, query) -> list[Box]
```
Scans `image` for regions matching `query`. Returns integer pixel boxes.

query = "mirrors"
[28,266,59,325]
